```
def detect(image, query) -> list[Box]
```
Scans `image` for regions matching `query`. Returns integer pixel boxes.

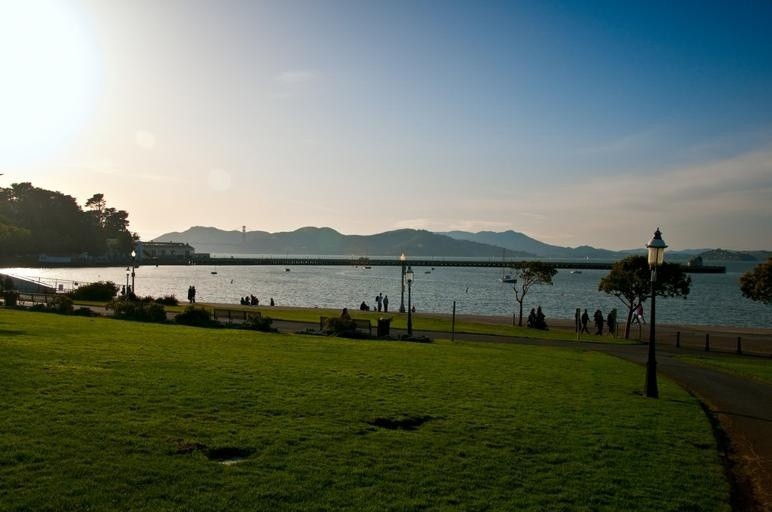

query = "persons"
[122,285,125,295]
[635,306,646,324]
[632,304,639,323]
[340,308,351,320]
[241,294,275,306]
[128,285,131,293]
[360,301,369,311]
[575,308,617,335]
[383,296,388,312]
[527,306,545,326]
[192,286,195,303]
[188,286,192,303]
[378,293,383,312]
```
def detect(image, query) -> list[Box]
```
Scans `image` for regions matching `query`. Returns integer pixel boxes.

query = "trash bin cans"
[59,284,63,291]
[377,318,391,337]
[3,290,18,305]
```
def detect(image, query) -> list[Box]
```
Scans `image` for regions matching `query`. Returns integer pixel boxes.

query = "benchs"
[333,318,372,333]
[214,308,261,322]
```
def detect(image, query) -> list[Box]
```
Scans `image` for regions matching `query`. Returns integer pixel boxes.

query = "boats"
[570,271,582,274]
[499,272,517,283]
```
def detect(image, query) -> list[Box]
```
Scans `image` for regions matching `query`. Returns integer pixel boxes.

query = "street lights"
[405,266,414,336]
[126,250,136,292]
[645,227,668,399]
[400,252,406,313]
